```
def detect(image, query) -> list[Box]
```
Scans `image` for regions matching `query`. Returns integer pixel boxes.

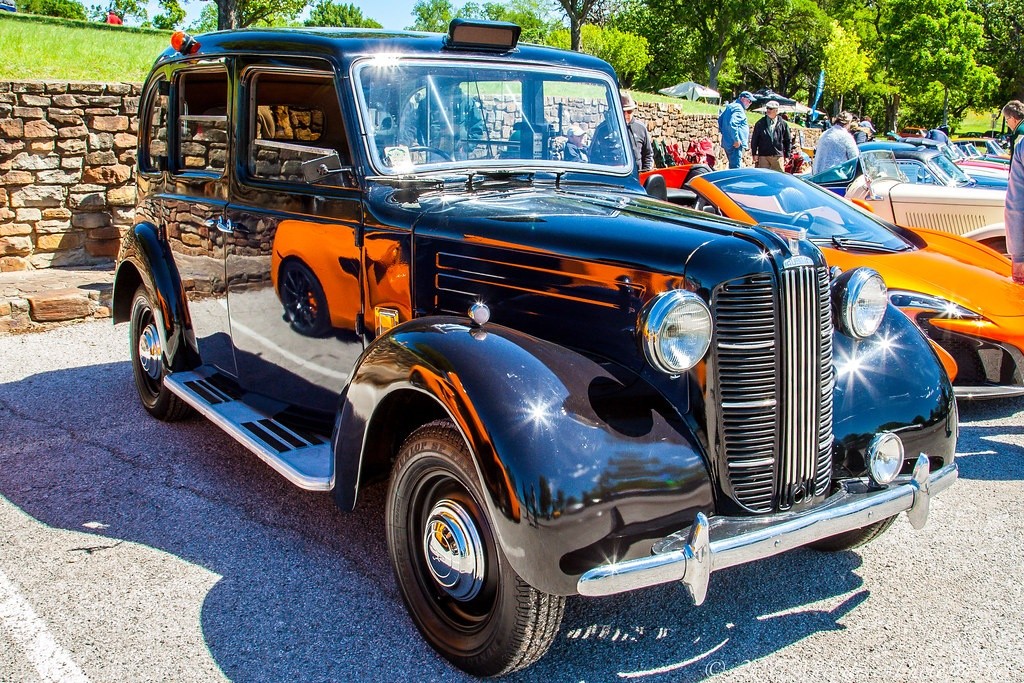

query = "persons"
[782,111,878,175]
[563,91,654,172]
[997,100,1024,288]
[717,90,753,169]
[925,124,951,147]
[373,77,485,162]
[750,100,792,172]
[106,10,123,25]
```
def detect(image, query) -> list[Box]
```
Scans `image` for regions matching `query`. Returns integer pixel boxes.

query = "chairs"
[642,174,667,201]
[693,197,722,217]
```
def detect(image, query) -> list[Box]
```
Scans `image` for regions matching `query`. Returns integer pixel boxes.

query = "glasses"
[746,98,752,103]
[1006,116,1013,122]
[766,107,776,110]
[622,109,635,114]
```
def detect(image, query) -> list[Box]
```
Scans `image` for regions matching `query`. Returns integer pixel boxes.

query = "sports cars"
[637,127,1024,402]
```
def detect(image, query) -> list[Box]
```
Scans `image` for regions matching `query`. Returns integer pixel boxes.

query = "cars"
[111,18,962,678]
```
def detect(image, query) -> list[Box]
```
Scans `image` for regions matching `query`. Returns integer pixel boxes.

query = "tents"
[750,86,827,128]
[658,81,722,106]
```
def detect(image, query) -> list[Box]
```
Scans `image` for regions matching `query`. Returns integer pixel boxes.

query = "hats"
[739,91,757,101]
[765,101,779,108]
[620,92,637,111]
[860,121,876,133]
[567,126,587,137]
